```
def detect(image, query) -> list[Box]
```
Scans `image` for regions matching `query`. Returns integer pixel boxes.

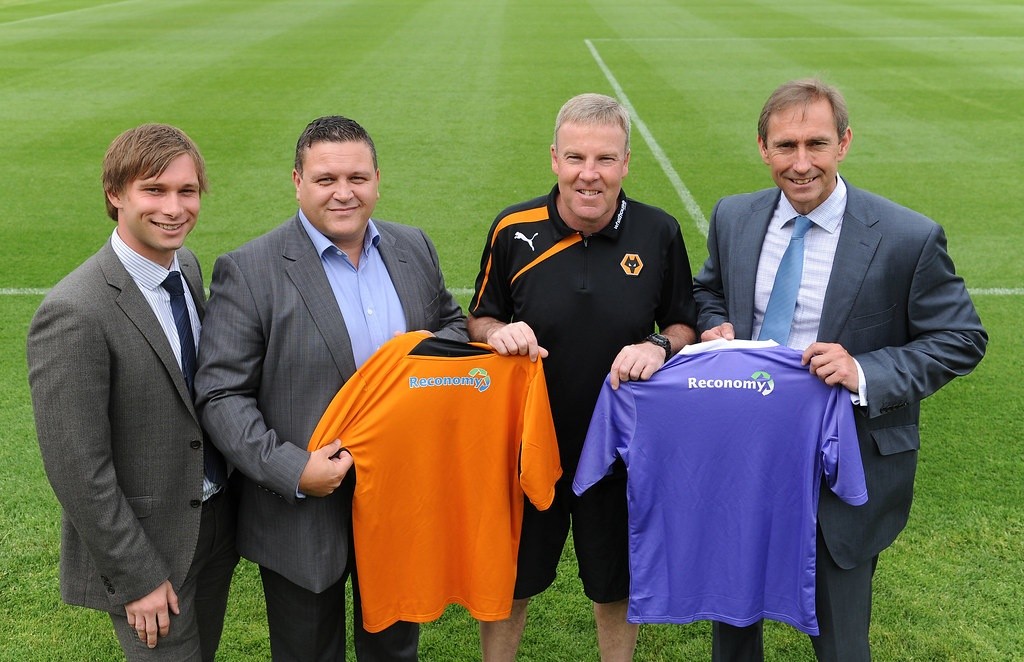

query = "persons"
[691,78,988,662]
[26,124,241,662]
[192,116,471,662]
[465,93,700,662]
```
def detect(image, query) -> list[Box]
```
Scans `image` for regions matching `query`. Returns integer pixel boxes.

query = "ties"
[160,271,229,488]
[758,215,817,347]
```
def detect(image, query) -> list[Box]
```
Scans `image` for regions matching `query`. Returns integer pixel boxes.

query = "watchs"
[642,333,671,363]
